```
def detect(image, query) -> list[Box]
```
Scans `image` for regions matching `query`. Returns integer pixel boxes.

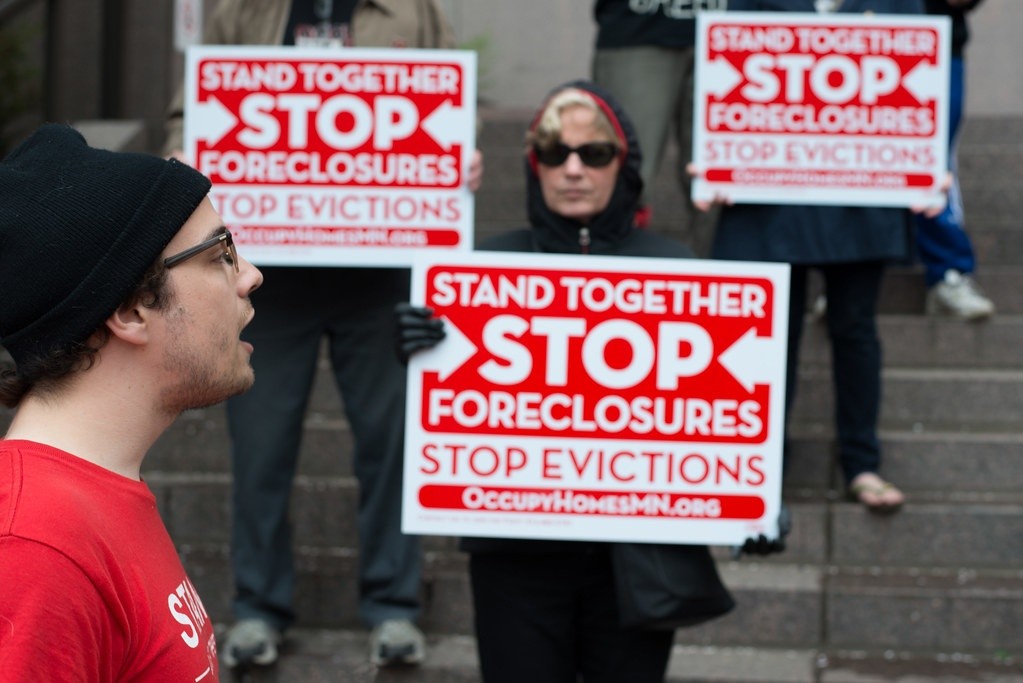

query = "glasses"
[160,230,238,275]
[535,140,623,168]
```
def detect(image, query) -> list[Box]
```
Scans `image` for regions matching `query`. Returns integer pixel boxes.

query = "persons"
[808,0,998,325]
[1,121,265,683]
[679,0,957,512]
[582,1,820,287]
[398,82,790,683]
[157,3,488,676]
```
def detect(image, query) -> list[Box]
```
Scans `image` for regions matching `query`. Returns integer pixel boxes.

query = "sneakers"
[927,277,994,320]
[375,619,427,666]
[220,614,283,669]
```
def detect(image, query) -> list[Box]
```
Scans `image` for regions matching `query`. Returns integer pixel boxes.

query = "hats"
[1,122,210,369]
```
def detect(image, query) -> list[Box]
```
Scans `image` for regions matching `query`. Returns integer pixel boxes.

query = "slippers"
[847,480,905,512]
